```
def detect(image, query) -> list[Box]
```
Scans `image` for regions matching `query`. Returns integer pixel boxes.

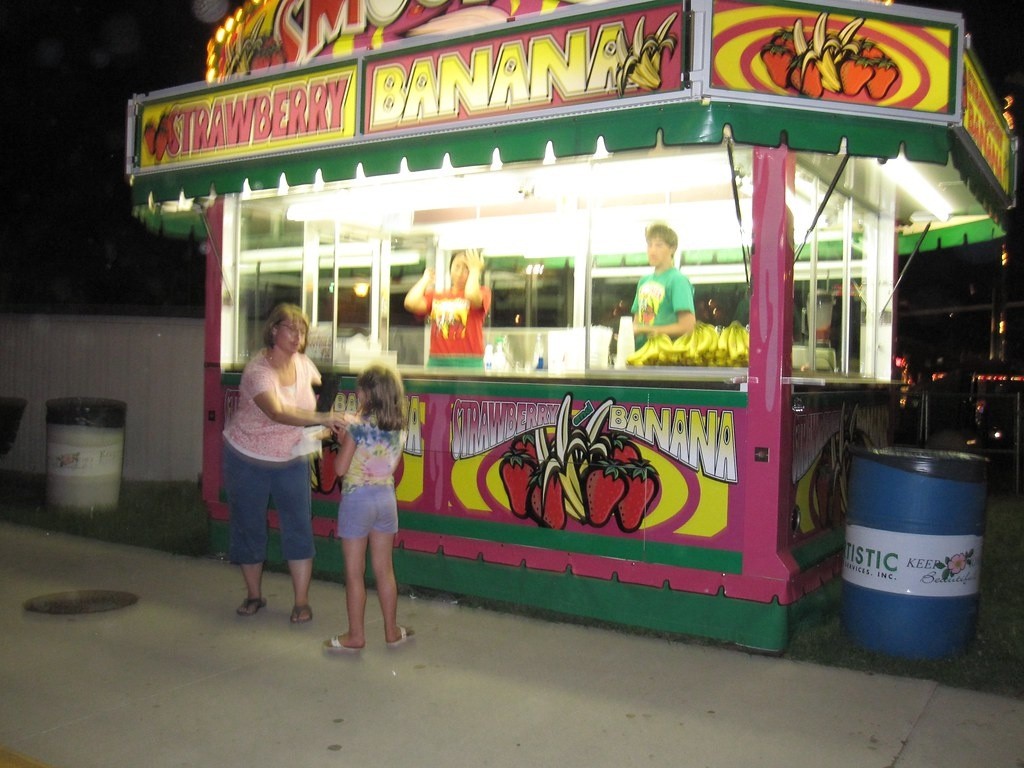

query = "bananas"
[624,320,749,368]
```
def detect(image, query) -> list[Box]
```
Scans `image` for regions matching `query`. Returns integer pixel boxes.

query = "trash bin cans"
[45,397,127,513]
[839,445,991,661]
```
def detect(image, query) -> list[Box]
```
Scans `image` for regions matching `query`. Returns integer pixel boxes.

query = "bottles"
[482,339,505,375]
[533,337,544,370]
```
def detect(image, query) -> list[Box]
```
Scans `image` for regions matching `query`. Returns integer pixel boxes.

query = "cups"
[615,316,636,368]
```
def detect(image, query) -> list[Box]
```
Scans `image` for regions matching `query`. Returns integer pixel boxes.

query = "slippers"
[324,633,360,654]
[290,603,312,624]
[237,598,261,616]
[386,624,416,647]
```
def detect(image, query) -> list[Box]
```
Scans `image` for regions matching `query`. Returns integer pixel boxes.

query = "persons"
[322,365,416,654]
[403,249,493,370]
[629,223,695,358]
[216,303,358,623]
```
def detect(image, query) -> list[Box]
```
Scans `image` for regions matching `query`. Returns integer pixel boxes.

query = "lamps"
[882,160,953,221]
